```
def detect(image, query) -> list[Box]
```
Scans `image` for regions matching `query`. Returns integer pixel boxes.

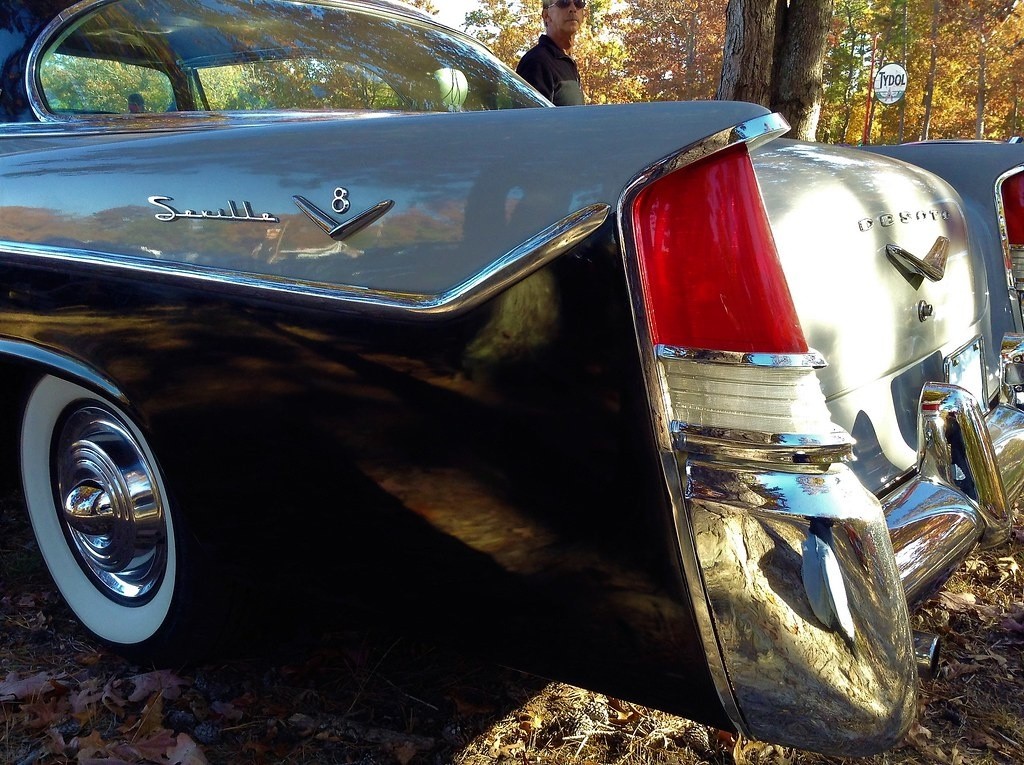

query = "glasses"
[546,0,586,10]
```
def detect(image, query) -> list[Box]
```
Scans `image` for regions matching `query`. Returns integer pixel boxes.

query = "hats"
[127,94,144,105]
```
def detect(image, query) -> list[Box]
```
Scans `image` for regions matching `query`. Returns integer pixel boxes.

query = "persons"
[515,0,586,106]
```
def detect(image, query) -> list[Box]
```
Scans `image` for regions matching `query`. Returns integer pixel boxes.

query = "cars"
[1,0,1024,756]
